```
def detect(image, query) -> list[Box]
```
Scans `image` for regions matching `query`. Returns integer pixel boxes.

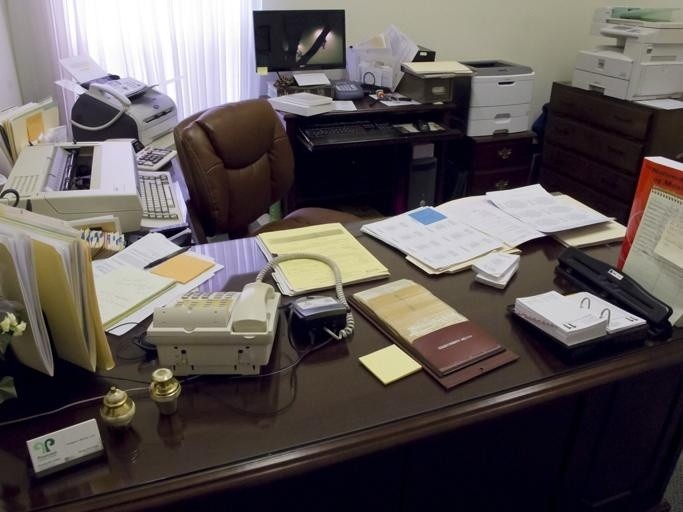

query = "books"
[402,61,473,75]
[92,232,216,332]
[617,156,683,330]
[256,222,391,297]
[1,96,62,169]
[551,221,628,248]
[267,92,335,119]
[0,202,117,377]
[345,276,520,395]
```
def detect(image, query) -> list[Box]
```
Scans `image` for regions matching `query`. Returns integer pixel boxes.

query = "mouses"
[412,119,430,134]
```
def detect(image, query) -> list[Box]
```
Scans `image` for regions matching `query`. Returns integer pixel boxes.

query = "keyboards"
[137,170,185,231]
[299,118,402,143]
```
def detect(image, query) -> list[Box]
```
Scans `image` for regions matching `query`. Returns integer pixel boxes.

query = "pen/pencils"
[144,246,191,269]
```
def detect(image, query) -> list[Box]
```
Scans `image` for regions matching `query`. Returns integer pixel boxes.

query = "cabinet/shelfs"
[446,81,683,227]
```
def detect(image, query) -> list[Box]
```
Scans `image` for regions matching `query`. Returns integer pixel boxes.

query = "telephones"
[144,282,281,377]
[89,82,131,113]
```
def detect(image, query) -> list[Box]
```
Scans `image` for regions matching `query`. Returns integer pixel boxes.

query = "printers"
[71,73,177,146]
[571,6,683,103]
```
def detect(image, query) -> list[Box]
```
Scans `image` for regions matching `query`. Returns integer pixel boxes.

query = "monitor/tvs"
[253,10,346,73]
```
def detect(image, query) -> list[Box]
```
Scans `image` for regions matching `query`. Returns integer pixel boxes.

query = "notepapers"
[471,251,520,289]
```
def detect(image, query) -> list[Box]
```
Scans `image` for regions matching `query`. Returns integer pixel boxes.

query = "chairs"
[173,98,361,240]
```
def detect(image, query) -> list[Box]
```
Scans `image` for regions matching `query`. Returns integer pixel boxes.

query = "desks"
[260,81,463,229]
